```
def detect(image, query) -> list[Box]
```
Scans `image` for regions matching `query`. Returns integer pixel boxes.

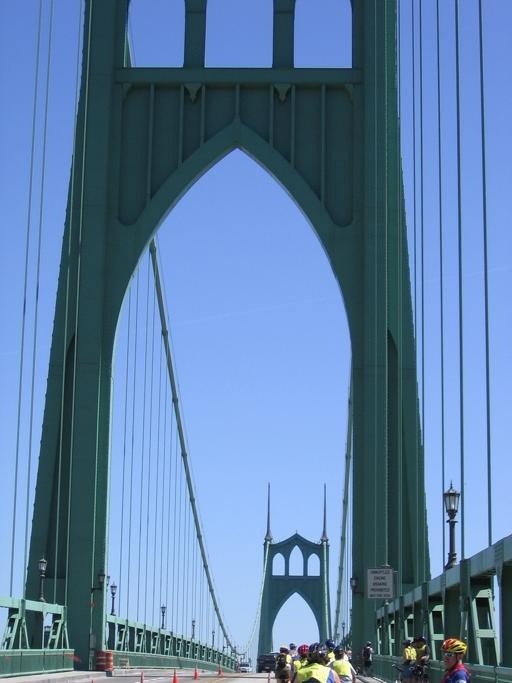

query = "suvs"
[256,652,277,673]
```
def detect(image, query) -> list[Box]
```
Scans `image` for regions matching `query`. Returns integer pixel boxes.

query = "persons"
[274,636,472,683]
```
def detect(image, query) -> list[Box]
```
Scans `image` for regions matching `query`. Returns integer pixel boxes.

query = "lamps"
[350,573,364,597]
[91,569,105,593]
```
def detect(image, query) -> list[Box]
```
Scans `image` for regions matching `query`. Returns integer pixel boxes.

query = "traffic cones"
[140,670,145,682]
[192,663,200,680]
[172,667,179,682]
[216,661,225,677]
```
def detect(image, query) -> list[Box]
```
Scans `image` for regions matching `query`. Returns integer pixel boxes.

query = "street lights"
[35,550,227,653]
[441,478,461,572]
[337,607,353,644]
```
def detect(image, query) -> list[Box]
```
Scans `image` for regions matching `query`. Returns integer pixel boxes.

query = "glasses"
[443,652,455,657]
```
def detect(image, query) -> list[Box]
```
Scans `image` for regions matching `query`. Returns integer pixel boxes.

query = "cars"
[238,661,252,672]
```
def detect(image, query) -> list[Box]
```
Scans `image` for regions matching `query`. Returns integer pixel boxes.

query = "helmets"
[278,639,345,662]
[401,635,427,648]
[440,637,468,654]
